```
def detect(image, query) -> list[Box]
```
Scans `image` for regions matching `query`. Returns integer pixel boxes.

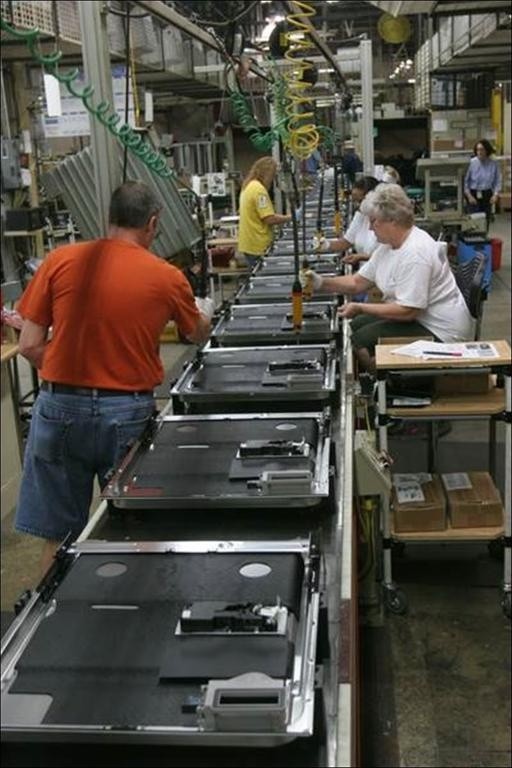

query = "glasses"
[475,148,482,151]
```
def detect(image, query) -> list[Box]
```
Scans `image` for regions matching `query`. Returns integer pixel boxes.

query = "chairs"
[428,221,443,240]
[448,242,488,341]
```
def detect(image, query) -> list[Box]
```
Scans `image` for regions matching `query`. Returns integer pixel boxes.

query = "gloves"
[299,269,322,292]
[313,236,330,252]
[192,291,219,323]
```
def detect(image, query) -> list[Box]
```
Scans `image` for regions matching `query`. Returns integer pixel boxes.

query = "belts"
[39,378,153,396]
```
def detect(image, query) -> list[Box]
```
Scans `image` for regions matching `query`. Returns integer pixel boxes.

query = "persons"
[296,182,479,431]
[232,158,299,293]
[461,138,504,233]
[311,174,390,301]
[14,181,219,624]
[303,137,363,186]
